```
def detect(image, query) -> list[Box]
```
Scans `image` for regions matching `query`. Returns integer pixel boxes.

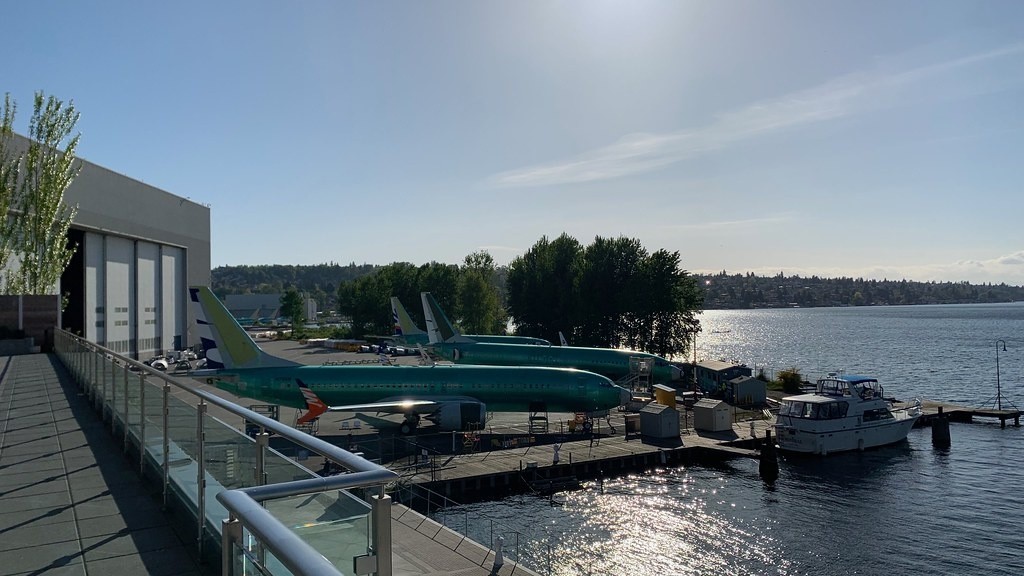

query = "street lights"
[996,339,1008,411]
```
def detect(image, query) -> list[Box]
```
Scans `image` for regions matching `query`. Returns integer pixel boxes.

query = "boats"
[774,374,922,454]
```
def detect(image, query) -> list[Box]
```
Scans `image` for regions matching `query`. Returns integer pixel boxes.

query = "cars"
[128,349,213,374]
[359,342,432,357]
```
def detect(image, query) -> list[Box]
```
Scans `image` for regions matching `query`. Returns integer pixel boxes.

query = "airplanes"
[187,286,687,440]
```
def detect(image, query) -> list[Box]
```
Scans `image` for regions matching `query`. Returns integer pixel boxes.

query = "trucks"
[244,403,282,440]
